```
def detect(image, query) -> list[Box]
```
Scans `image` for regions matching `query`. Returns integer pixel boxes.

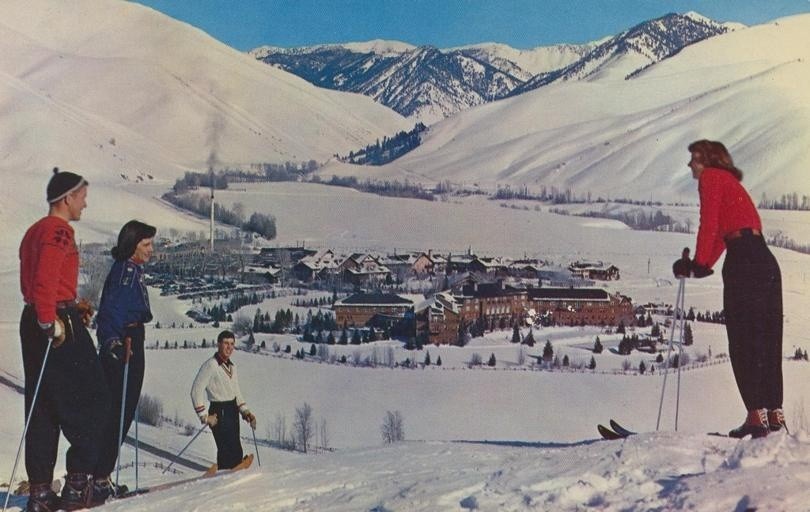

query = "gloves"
[242,410,257,429]
[75,298,93,326]
[201,415,218,426]
[109,341,136,364]
[48,319,66,349]
[673,259,714,278]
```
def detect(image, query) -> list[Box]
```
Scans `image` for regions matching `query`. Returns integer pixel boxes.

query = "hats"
[47,166,85,204]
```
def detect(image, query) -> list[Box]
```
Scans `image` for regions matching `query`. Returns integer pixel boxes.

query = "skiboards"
[598,420,793,440]
[204,454,254,479]
[19,466,207,512]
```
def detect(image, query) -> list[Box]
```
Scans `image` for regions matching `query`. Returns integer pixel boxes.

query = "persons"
[673,139,788,439]
[88,220,159,503]
[17,168,113,511]
[189,331,256,472]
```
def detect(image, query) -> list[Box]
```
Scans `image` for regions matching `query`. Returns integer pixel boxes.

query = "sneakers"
[767,408,784,430]
[27,484,62,512]
[61,472,127,511]
[729,409,768,438]
